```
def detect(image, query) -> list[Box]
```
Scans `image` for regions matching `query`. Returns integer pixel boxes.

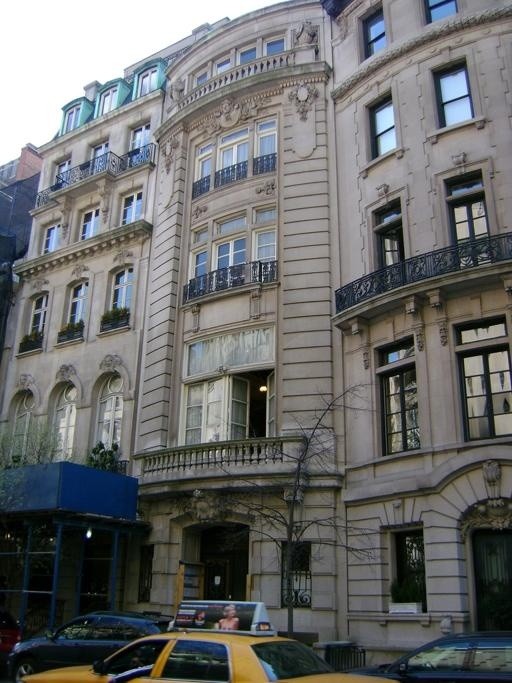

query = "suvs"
[3,606,173,682]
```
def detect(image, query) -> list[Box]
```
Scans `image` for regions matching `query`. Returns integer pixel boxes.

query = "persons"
[215,604,241,631]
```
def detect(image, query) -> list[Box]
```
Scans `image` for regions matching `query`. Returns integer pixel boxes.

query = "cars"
[0,606,22,659]
[18,600,399,682]
[338,631,512,682]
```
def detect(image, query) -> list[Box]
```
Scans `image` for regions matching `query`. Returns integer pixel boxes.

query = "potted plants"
[389,573,425,613]
